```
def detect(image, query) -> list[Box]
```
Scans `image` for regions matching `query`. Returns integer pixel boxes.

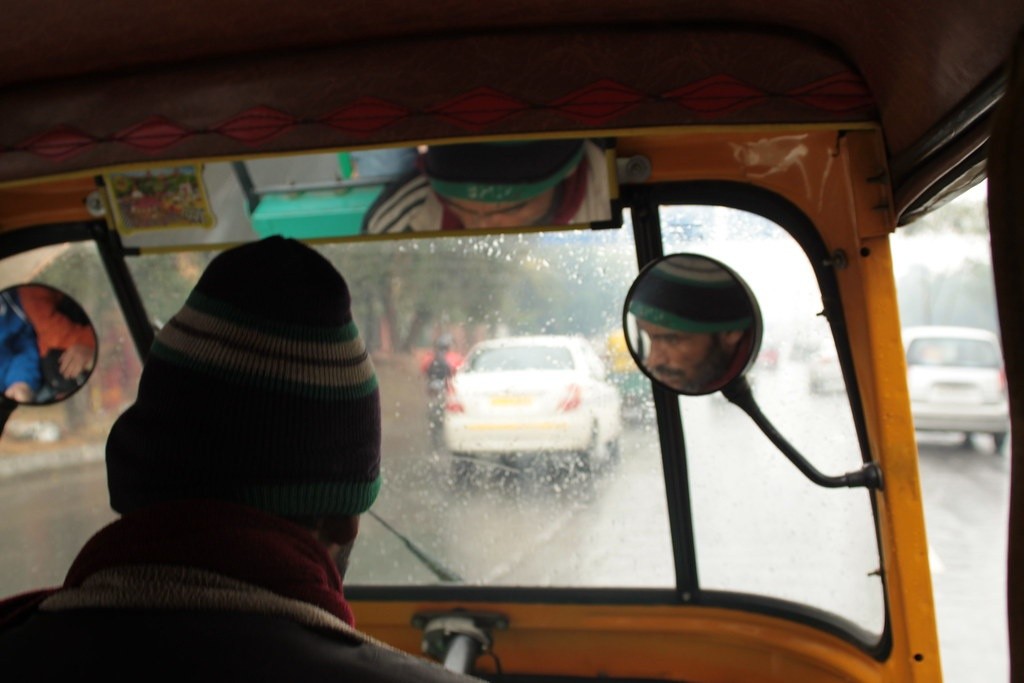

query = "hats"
[419,143,588,204]
[135,235,382,517]
[630,252,758,332]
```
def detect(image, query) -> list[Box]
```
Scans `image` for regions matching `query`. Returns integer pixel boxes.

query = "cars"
[809,338,849,396]
[900,324,1009,454]
[442,335,624,478]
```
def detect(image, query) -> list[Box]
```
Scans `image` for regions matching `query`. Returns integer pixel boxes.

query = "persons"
[362,138,612,236]
[610,321,637,375]
[629,257,753,394]
[0,285,97,403]
[423,335,461,380]
[0,236,488,683]
